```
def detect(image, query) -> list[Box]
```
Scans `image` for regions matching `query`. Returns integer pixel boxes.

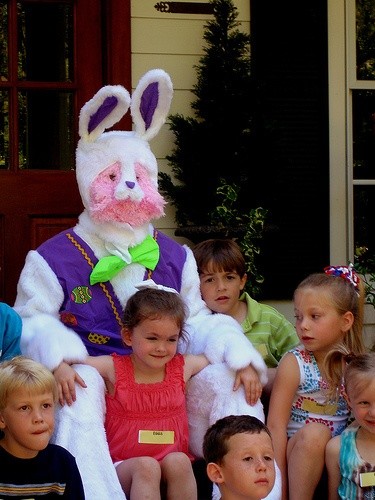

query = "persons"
[322,341,375,500]
[266,266,375,500]
[202,415,276,500]
[0,355,87,500]
[50,279,263,500]
[167,239,308,464]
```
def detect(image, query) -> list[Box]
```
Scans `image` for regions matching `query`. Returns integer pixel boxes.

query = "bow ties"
[90,234,160,285]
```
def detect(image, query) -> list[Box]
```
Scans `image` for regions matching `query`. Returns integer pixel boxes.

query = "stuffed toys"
[7,68,283,499]
